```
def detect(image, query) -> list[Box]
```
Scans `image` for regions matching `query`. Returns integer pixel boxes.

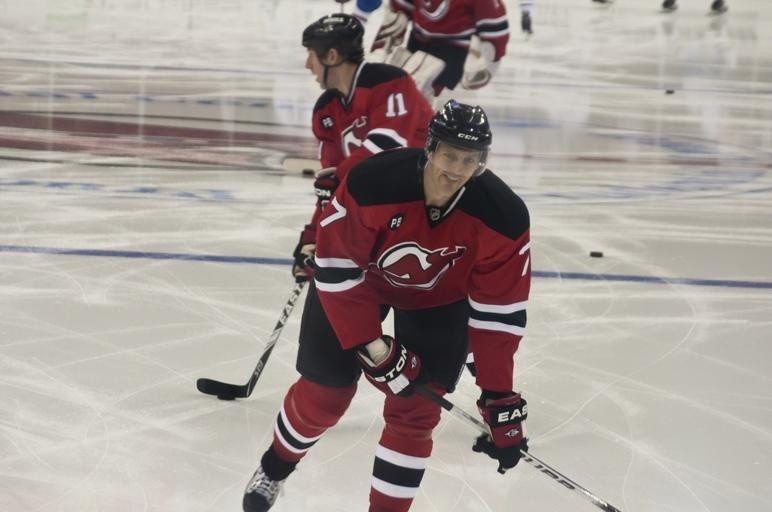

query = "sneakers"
[243,464,287,512]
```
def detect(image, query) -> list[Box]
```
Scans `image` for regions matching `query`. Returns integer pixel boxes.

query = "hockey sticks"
[196,275,305,400]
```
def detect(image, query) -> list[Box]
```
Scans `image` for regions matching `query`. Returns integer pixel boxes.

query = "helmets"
[303,14,366,58]
[425,99,492,152]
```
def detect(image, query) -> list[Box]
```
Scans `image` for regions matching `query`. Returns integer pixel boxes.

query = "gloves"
[473,393,530,472]
[313,166,341,215]
[353,335,423,398]
[292,224,318,284]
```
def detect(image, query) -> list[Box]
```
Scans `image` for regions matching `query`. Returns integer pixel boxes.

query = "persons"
[240,98,534,511]
[658,1,731,17]
[336,1,513,109]
[289,11,442,287]
